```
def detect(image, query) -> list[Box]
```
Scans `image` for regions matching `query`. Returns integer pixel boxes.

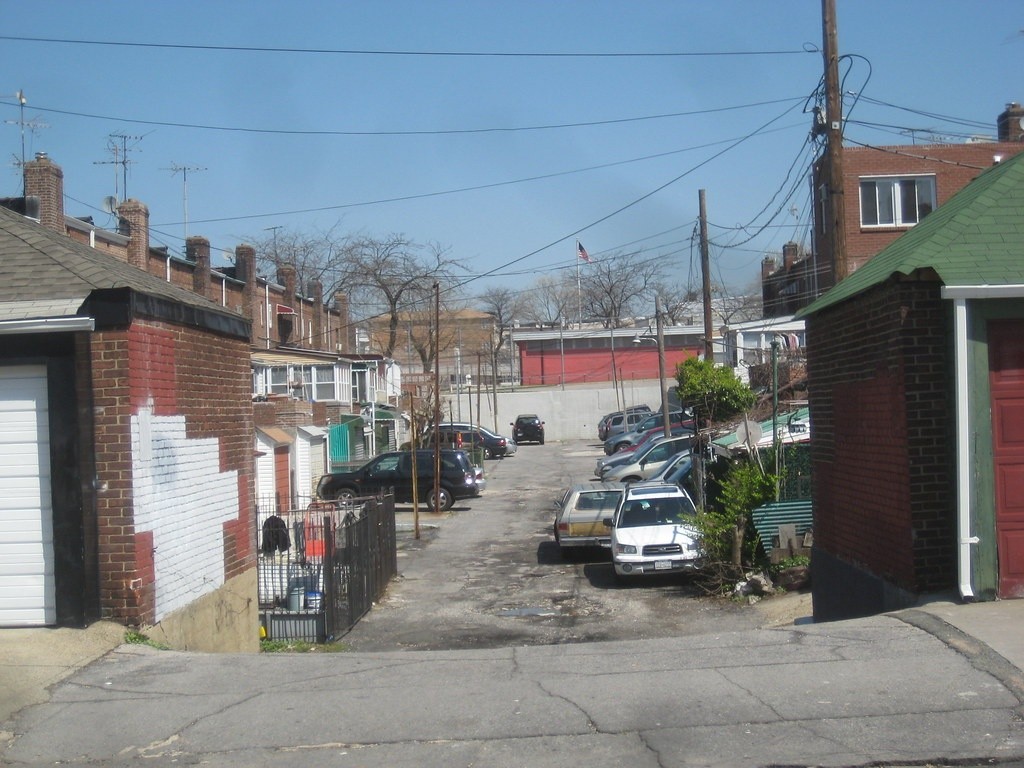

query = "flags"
[579,242,592,264]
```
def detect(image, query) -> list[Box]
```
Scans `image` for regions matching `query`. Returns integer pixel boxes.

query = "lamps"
[789,412,807,433]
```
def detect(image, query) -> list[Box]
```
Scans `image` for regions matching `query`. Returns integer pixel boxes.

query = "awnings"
[372,409,402,420]
[341,414,372,427]
[361,402,393,408]
[276,304,298,315]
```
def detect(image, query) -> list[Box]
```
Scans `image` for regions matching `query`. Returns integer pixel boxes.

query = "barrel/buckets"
[286,586,304,611]
[307,592,321,609]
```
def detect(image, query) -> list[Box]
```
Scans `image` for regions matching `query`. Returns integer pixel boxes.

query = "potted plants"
[776,554,811,589]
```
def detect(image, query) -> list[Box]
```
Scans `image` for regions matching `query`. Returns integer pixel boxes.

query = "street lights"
[16,90,26,163]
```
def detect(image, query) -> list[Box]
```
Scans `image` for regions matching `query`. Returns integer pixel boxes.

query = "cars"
[510,414,545,445]
[316,450,479,512]
[399,422,517,460]
[555,403,710,586]
